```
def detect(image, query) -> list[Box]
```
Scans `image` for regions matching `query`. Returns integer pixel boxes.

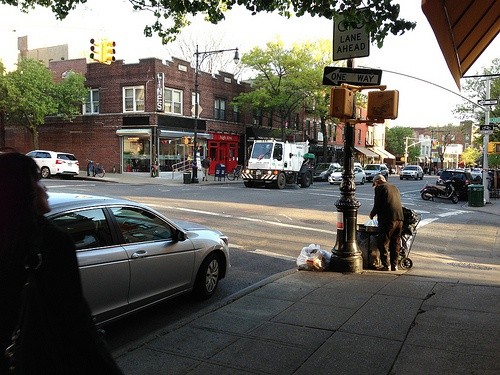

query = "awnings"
[421,0,500,91]
[355,147,395,159]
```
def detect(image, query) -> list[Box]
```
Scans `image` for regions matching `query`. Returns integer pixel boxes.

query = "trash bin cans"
[468,184,484,206]
[355,222,383,268]
[214,164,225,182]
[183,173,191,183]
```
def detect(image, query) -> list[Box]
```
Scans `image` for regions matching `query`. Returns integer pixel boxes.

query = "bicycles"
[86,159,106,178]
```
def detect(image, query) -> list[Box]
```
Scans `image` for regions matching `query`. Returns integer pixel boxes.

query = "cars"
[314,163,342,182]
[399,165,424,180]
[354,163,363,168]
[364,164,390,182]
[328,167,366,185]
[26,150,80,180]
[441,167,500,194]
[43,192,231,329]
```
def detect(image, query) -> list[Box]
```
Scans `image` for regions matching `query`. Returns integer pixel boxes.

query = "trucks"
[242,138,315,190]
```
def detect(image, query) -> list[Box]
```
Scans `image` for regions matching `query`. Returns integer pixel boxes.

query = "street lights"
[191,44,239,184]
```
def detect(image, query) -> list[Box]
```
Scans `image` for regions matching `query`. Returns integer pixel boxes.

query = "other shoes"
[392,266,398,271]
[380,266,391,271]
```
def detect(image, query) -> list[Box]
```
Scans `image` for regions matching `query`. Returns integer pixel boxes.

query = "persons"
[0,151,124,375]
[202,156,211,181]
[370,174,403,271]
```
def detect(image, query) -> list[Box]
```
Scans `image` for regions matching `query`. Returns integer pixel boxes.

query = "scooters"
[421,176,459,204]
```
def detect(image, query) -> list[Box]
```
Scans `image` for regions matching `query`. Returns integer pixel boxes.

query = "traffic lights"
[89,39,103,62]
[495,142,500,153]
[104,39,116,65]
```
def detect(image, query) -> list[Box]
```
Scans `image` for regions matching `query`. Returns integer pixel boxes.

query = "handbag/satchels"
[6,223,87,375]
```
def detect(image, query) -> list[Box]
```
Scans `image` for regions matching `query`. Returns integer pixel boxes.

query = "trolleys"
[399,207,422,270]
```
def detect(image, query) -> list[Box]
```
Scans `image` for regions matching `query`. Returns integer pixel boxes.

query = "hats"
[372,174,384,186]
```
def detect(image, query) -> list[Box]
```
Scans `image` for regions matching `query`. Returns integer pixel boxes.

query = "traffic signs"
[477,99,498,106]
[323,66,382,86]
